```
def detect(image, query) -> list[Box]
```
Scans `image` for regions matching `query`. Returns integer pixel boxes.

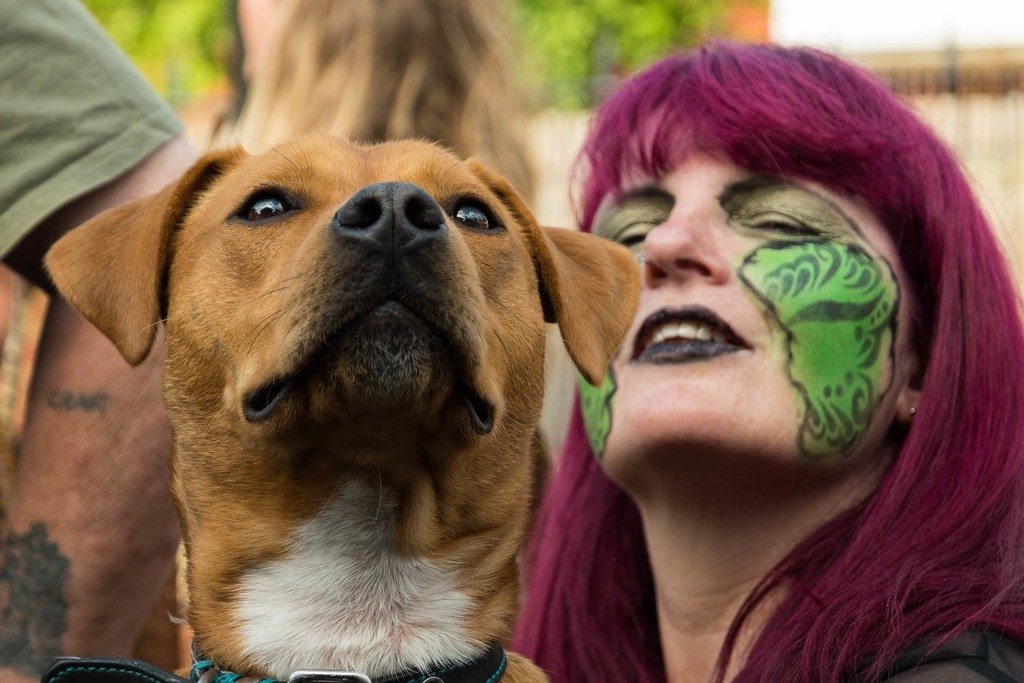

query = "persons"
[0,0,208,683]
[498,41,1024,683]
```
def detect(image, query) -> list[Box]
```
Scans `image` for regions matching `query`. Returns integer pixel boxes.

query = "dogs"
[42,134,642,683]
